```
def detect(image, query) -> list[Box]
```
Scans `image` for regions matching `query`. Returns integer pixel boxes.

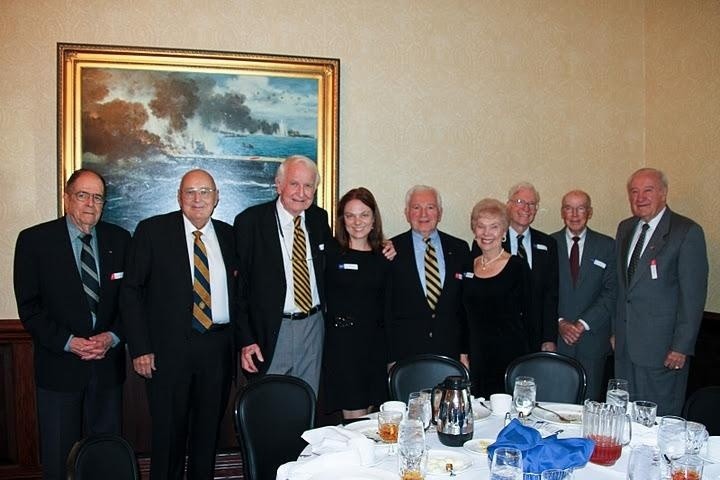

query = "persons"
[387,185,470,373]
[318,187,392,427]
[550,191,615,403]
[456,199,536,400]
[233,155,398,397]
[120,170,235,479]
[611,168,710,418]
[14,169,133,479]
[471,182,560,354]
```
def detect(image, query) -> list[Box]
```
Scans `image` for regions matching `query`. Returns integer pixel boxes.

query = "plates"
[471,402,491,422]
[427,439,496,478]
[532,403,585,426]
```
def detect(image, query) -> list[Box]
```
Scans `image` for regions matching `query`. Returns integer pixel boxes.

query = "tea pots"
[430,374,476,448]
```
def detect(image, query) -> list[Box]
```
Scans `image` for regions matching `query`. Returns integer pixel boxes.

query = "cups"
[584,377,707,480]
[491,446,574,480]
[489,375,537,416]
[377,388,431,480]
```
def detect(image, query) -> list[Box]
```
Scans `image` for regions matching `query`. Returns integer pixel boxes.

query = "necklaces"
[481,248,505,272]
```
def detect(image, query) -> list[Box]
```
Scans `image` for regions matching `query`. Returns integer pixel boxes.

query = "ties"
[516,235,527,258]
[191,231,213,334]
[569,237,580,288]
[78,232,100,317]
[422,237,442,311]
[292,215,312,314]
[628,224,649,286]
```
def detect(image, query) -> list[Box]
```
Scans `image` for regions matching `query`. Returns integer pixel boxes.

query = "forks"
[535,404,577,422]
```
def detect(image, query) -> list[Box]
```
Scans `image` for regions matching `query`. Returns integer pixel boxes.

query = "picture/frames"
[56,42,340,238]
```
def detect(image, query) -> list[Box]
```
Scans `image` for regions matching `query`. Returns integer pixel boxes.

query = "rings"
[675,365,680,371]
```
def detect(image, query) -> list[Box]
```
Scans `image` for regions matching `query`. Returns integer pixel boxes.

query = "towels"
[276,425,398,480]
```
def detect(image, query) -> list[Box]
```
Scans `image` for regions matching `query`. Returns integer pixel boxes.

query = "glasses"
[76,191,104,204]
[509,199,537,209]
[184,188,216,198]
[282,237,318,262]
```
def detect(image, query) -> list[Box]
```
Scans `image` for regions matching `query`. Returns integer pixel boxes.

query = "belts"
[283,304,322,320]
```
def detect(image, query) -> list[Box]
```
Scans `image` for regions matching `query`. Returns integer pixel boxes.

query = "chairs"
[66,431,140,480]
[505,351,587,405]
[234,374,318,480]
[387,354,473,411]
